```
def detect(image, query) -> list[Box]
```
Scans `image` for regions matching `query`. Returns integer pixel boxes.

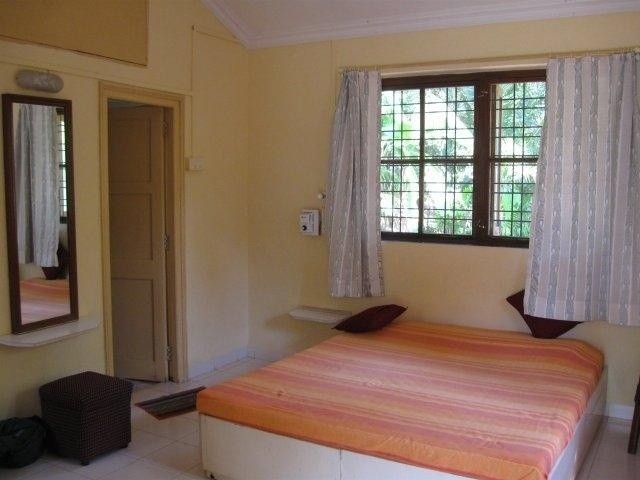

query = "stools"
[39,370,132,466]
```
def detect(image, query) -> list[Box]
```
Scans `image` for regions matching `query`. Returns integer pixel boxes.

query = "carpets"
[135,385,208,420]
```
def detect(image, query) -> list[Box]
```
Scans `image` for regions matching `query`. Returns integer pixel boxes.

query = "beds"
[19,277,70,325]
[196,321,609,480]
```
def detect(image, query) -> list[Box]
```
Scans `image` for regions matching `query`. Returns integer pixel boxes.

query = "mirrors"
[1,93,80,335]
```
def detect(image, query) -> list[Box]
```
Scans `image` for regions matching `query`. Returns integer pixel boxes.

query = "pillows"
[504,288,583,338]
[332,303,408,333]
[42,243,69,280]
